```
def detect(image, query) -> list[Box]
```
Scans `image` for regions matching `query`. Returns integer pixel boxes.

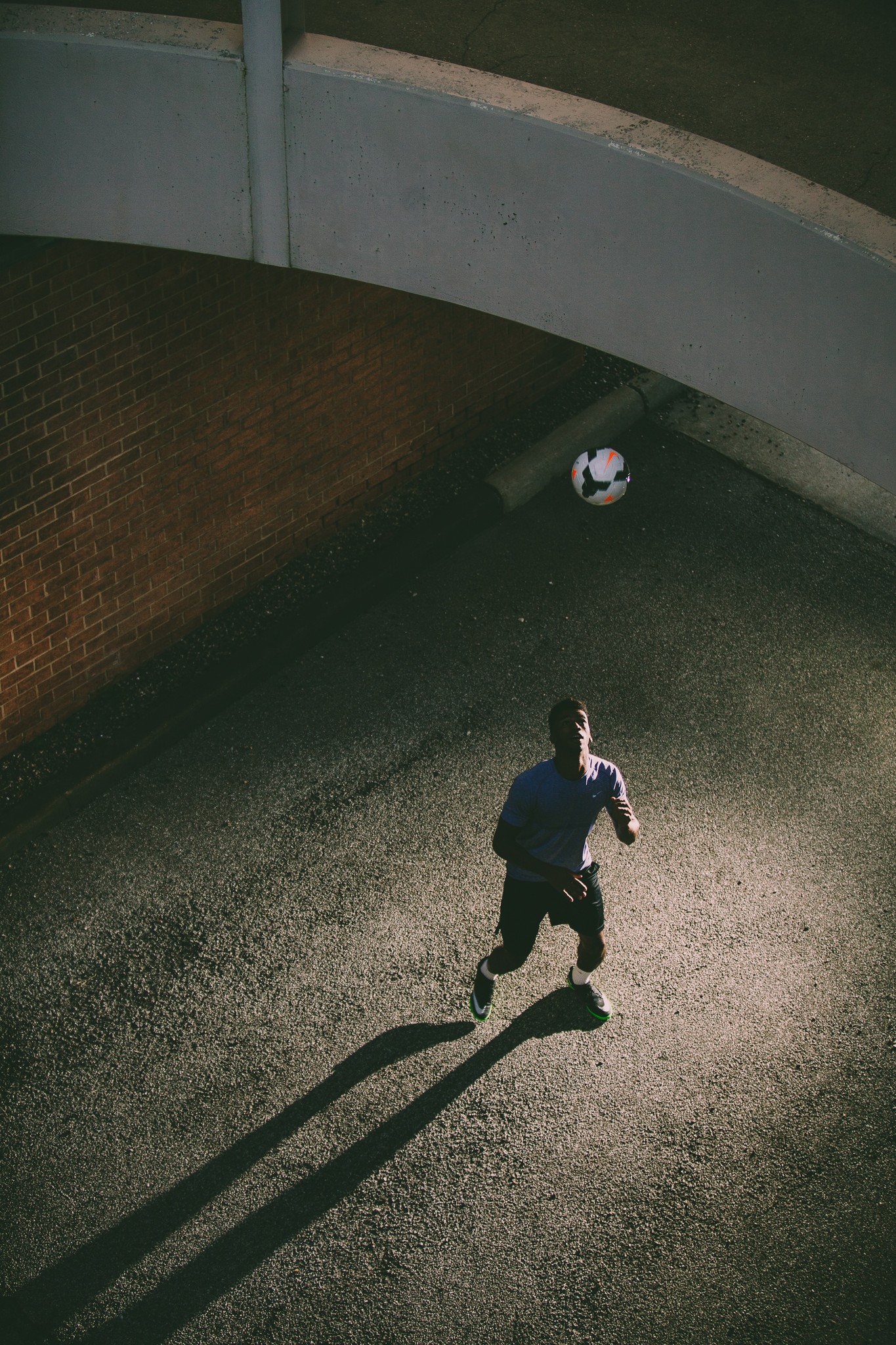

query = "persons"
[470,696,640,1023]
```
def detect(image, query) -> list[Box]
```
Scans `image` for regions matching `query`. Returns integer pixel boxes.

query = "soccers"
[570,446,632,507]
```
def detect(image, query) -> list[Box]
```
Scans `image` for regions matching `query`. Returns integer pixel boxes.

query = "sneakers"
[469,957,497,1023]
[566,966,612,1020]
